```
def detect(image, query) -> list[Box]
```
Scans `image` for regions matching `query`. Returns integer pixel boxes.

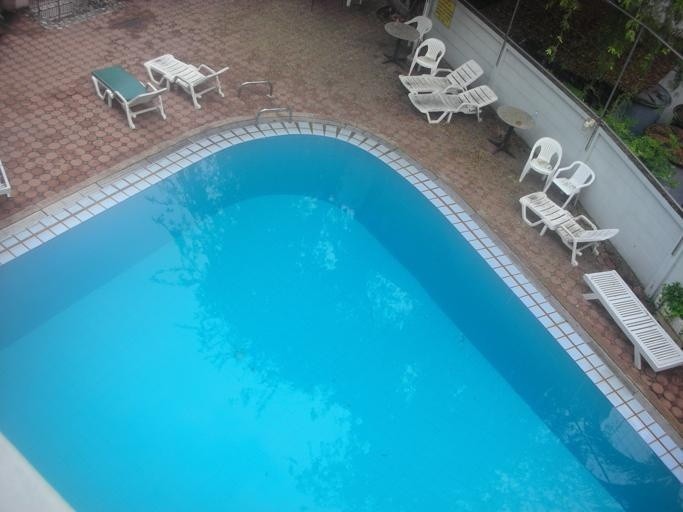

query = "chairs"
[89,53,230,130]
[518,136,620,267]
[399,13,498,125]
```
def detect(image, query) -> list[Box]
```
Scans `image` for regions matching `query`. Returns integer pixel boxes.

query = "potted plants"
[653,282,683,335]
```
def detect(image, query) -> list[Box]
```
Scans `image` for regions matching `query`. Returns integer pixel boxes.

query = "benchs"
[580,270,683,375]
